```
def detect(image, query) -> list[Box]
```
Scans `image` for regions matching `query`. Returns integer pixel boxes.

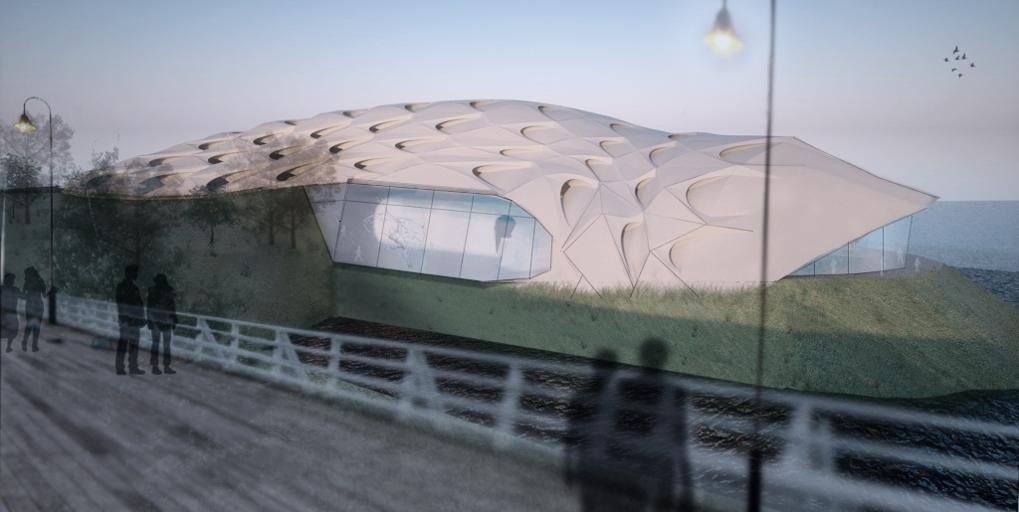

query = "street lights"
[15,95,58,325]
[704,0,777,510]
